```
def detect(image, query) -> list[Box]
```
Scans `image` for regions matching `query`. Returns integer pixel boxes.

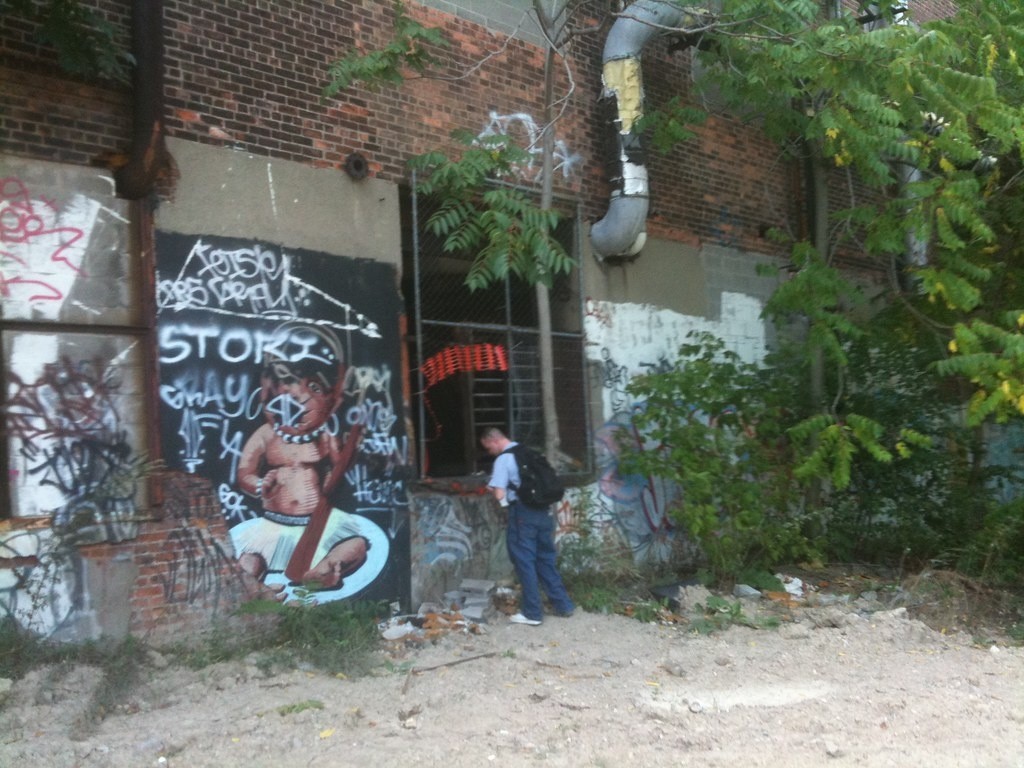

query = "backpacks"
[497,444,565,509]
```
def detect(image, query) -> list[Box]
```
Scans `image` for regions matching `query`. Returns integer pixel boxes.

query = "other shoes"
[544,604,572,617]
[509,612,542,625]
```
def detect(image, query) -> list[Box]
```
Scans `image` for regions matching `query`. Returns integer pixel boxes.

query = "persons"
[481,425,575,625]
